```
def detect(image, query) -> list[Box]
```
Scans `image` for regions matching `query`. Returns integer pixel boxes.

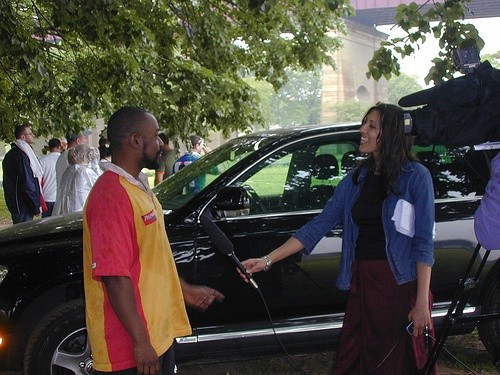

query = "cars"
[0,120,500,375]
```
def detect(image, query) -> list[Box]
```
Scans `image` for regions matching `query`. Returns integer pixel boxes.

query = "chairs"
[412,151,442,178]
[307,149,361,210]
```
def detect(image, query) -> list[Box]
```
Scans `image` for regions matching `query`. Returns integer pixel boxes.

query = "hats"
[65,126,93,143]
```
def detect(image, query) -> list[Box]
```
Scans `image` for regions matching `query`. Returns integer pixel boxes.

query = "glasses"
[22,133,33,136]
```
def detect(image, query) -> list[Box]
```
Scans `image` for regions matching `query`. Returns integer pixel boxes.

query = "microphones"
[200,214,260,291]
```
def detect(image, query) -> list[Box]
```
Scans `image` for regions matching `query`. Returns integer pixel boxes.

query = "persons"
[52,144,103,218]
[59,140,68,154]
[235,102,439,375]
[2,125,45,225]
[97,136,113,170]
[173,134,220,194]
[81,105,225,375]
[56,128,92,196]
[155,132,176,185]
[38,137,63,218]
[473,150,500,249]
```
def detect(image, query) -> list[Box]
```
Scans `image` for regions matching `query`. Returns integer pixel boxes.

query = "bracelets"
[262,255,272,271]
